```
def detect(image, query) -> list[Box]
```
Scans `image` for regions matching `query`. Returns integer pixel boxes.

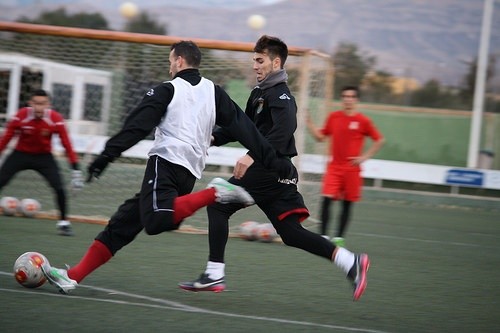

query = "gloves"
[69,168,84,189]
[85,147,115,183]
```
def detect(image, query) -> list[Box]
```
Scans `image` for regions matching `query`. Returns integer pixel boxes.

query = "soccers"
[239,221,276,242]
[14,252,50,288]
[0,196,41,218]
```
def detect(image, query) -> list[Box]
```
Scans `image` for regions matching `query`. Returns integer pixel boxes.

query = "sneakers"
[177,273,225,293]
[206,178,255,207]
[346,252,369,301]
[40,264,79,295]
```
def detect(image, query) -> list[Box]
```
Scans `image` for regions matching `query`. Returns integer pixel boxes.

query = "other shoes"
[330,236,344,248]
[56,220,72,235]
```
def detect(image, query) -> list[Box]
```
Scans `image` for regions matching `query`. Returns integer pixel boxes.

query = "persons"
[0,90,84,236]
[306,86,386,246]
[40,42,292,295]
[178,35,370,301]
